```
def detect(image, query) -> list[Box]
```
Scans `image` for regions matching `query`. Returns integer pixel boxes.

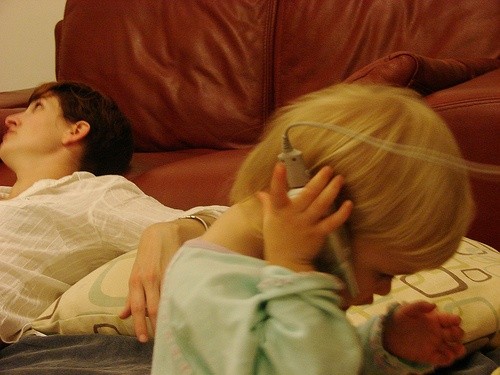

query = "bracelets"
[177,216,209,231]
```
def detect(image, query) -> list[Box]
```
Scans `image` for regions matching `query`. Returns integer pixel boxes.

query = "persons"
[150,83,476,375]
[0,82,230,347]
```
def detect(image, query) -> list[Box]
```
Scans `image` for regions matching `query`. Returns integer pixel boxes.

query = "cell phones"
[289,188,360,299]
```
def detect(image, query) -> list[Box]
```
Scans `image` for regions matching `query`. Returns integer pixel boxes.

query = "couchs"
[0,0,500,248]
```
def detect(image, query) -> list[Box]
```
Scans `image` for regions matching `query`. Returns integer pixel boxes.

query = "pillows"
[346,236,500,352]
[347,51,469,99]
[31,250,157,336]
